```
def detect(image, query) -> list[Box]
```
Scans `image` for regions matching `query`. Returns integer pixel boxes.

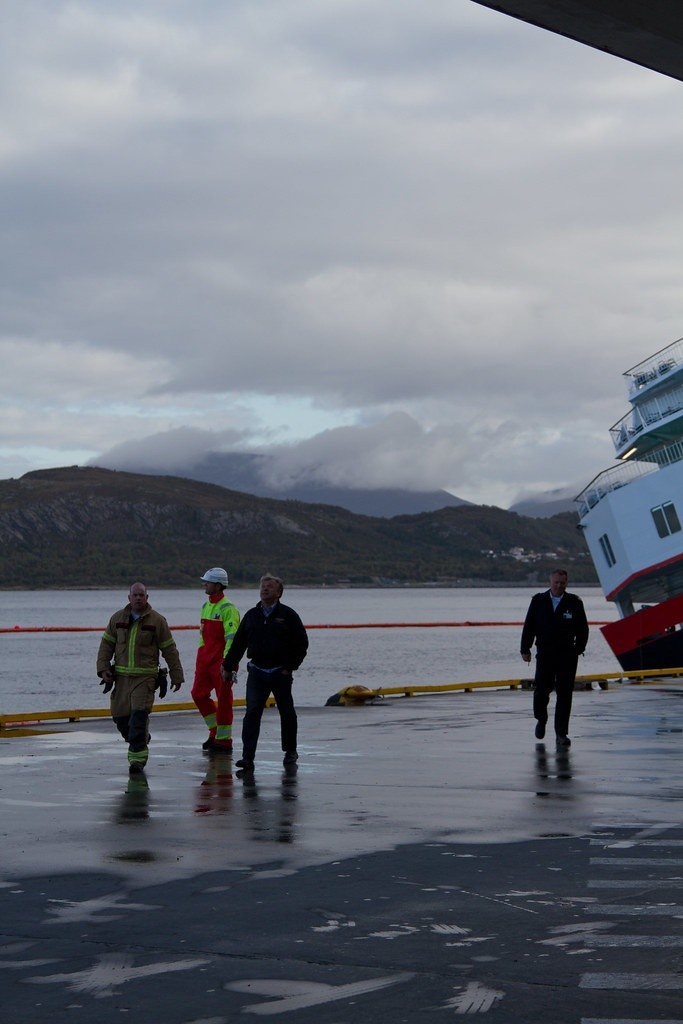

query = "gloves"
[100,666,114,693]
[153,667,167,699]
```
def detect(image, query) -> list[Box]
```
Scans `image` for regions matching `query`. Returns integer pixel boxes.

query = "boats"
[572,338,683,684]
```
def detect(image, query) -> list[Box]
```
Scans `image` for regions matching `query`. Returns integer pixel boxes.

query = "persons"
[191,567,240,753]
[221,576,308,771]
[520,569,589,747]
[97,582,185,772]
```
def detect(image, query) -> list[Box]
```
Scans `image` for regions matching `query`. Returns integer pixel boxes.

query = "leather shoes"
[127,760,143,773]
[200,738,214,749]
[555,734,571,746]
[535,718,547,740]
[234,758,253,769]
[207,743,232,755]
[283,759,298,774]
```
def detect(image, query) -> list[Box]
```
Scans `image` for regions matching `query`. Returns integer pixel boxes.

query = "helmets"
[200,567,229,587]
